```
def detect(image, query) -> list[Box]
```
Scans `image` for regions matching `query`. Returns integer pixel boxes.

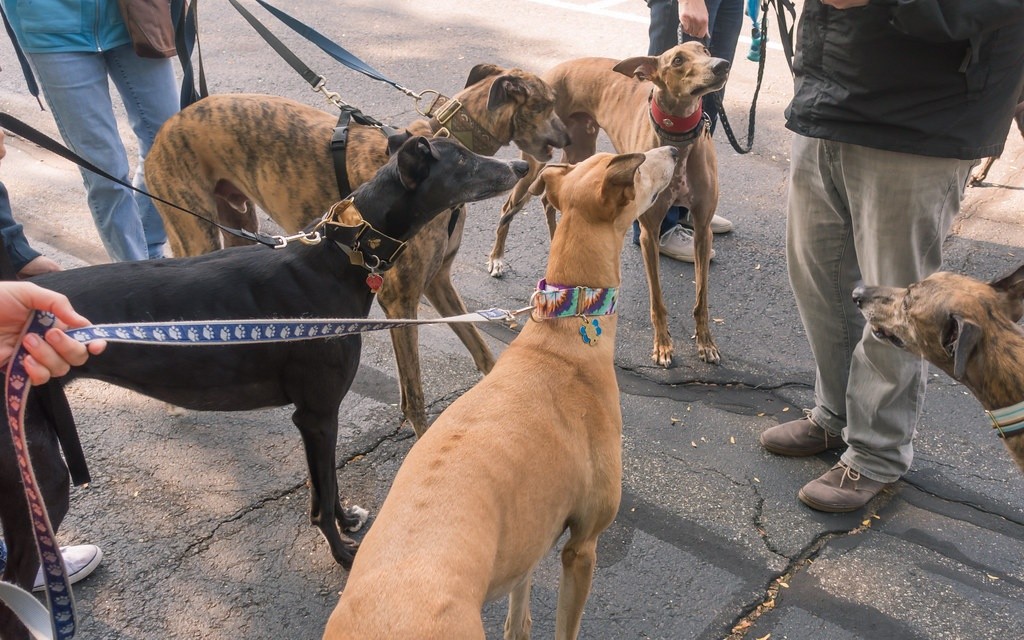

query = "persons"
[632,0,743,263]
[760,0,1024,514]
[0,0,181,263]
[0,130,103,640]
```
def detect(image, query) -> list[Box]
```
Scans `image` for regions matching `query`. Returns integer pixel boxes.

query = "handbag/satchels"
[118,0,194,59]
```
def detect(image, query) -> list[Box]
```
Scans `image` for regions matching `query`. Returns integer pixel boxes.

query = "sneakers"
[660,223,715,262]
[799,459,885,514]
[760,408,848,457]
[678,208,732,233]
[32,544,102,592]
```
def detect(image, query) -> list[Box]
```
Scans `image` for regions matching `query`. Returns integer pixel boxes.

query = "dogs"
[1,130,530,639]
[320,144,682,639]
[485,39,729,366]
[850,267,1024,472]
[144,64,573,442]
